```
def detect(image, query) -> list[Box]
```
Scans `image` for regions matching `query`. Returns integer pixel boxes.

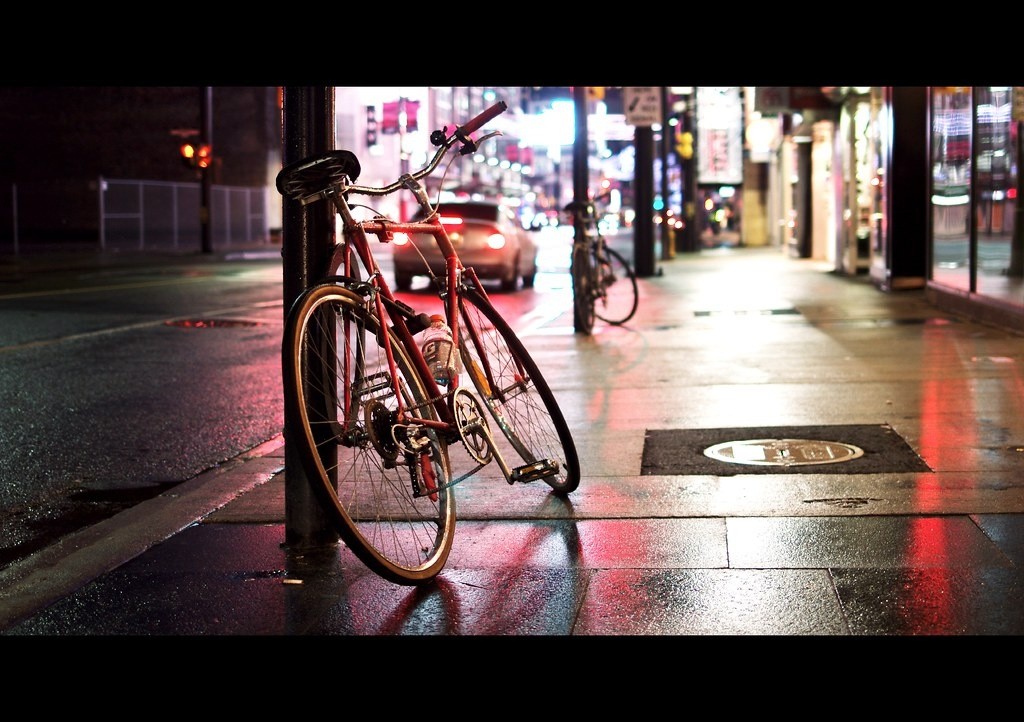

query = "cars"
[391,199,540,296]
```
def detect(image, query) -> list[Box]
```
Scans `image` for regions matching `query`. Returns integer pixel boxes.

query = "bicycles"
[276,100,581,589]
[560,187,640,337]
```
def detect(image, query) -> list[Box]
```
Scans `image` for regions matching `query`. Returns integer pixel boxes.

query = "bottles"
[422,314,456,383]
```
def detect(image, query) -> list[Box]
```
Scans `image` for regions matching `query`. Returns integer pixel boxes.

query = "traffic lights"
[174,130,213,177]
